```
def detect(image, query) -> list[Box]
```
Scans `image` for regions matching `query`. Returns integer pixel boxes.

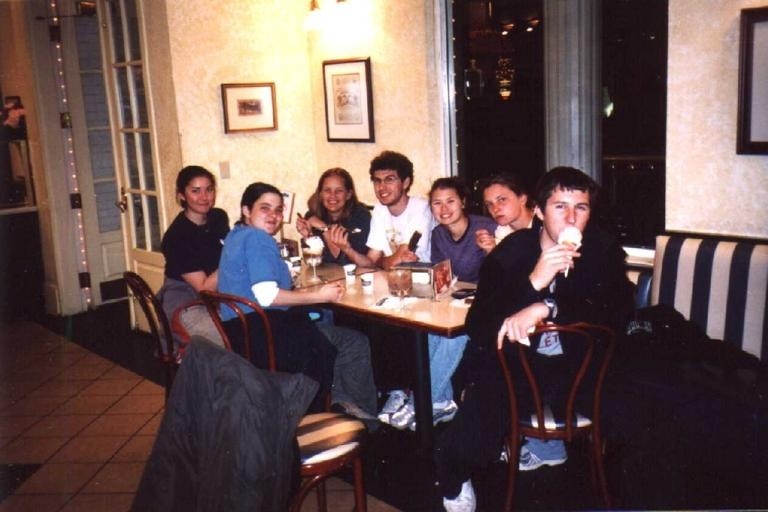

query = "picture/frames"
[217,79,282,136]
[732,3,768,158]
[320,54,378,145]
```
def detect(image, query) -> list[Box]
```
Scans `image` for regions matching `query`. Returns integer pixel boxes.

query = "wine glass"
[298,235,323,283]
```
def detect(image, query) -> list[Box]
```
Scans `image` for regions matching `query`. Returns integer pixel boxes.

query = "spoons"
[319,226,360,233]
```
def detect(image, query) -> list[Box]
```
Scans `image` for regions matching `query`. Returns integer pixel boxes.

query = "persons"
[428,177,497,284]
[161,163,228,354]
[442,166,633,511]
[296,167,371,270]
[473,172,538,256]
[218,181,378,432]
[330,152,436,271]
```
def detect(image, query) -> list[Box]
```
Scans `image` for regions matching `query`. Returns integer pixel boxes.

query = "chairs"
[494,322,620,511]
[199,287,372,510]
[121,269,208,422]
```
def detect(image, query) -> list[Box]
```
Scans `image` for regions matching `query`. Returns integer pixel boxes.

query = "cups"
[289,255,301,274]
[359,271,373,295]
[387,265,413,298]
[342,263,356,285]
[279,243,290,260]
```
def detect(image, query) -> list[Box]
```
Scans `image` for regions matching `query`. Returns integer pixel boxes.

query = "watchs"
[541,298,558,320]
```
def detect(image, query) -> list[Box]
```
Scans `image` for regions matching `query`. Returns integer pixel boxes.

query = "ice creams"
[494,225,514,247]
[558,227,583,279]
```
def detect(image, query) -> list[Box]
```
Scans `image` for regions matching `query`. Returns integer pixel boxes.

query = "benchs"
[617,234,768,479]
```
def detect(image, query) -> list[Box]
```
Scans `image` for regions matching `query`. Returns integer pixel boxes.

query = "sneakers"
[377,390,458,432]
[517,439,567,471]
